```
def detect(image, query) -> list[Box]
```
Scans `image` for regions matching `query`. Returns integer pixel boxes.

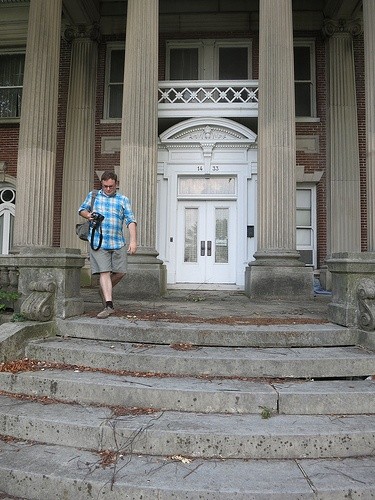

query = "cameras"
[91,212,104,229]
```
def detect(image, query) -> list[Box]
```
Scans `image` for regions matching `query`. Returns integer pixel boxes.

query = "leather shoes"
[99,287,107,307]
[97,307,116,319]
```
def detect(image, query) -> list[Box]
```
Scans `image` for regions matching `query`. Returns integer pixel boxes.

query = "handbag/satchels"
[76,190,99,241]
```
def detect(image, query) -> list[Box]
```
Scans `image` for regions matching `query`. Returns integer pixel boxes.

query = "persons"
[77,171,137,319]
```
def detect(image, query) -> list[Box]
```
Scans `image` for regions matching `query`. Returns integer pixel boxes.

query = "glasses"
[103,185,116,189]
